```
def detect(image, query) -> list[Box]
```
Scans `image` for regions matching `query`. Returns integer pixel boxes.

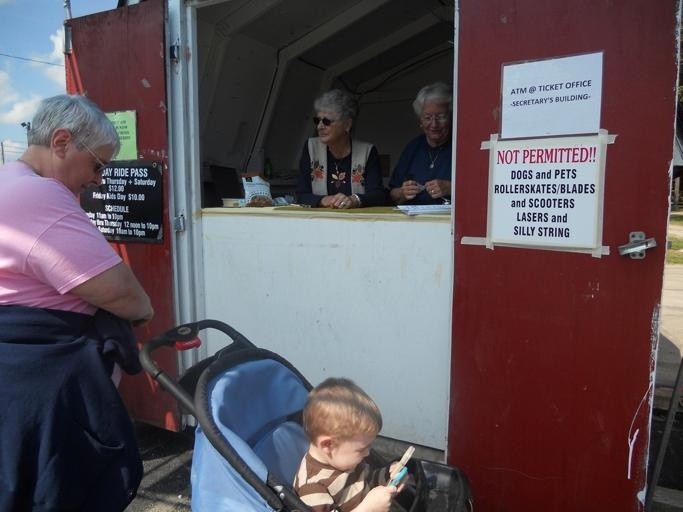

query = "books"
[397,204,451,217]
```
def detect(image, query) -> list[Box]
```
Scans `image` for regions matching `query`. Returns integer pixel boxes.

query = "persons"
[2,94,154,511]
[293,376,407,511]
[387,80,453,203]
[249,87,390,206]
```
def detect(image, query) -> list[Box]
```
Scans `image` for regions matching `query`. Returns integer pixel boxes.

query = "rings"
[431,188,434,195]
[342,201,345,206]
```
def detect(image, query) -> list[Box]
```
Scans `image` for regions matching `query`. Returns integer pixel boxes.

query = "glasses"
[313,116,345,126]
[72,133,111,173]
[419,111,451,122]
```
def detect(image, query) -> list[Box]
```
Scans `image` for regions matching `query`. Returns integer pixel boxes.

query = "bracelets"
[352,194,362,208]
[399,188,406,201]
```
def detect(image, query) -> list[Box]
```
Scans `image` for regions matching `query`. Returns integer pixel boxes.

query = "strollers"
[137,319,476,512]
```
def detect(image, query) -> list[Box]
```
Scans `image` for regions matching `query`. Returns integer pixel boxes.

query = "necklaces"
[426,142,443,170]
[327,144,351,170]
